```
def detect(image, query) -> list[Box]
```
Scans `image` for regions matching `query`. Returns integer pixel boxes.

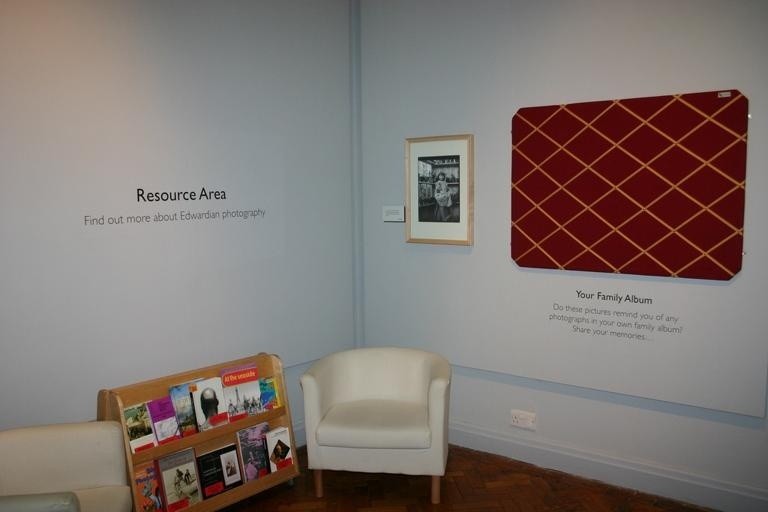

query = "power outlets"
[510,407,538,431]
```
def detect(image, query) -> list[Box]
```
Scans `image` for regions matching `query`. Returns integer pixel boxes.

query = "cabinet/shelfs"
[96,351,301,512]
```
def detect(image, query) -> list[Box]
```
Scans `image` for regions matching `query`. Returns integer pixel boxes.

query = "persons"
[433,171,454,222]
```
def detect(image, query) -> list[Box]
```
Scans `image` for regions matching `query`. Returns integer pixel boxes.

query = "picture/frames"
[403,133,475,246]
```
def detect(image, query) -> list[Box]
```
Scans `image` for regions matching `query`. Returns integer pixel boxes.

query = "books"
[123,359,295,511]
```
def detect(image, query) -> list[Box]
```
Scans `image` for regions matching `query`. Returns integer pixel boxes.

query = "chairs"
[297,345,452,505]
[0,421,135,511]
